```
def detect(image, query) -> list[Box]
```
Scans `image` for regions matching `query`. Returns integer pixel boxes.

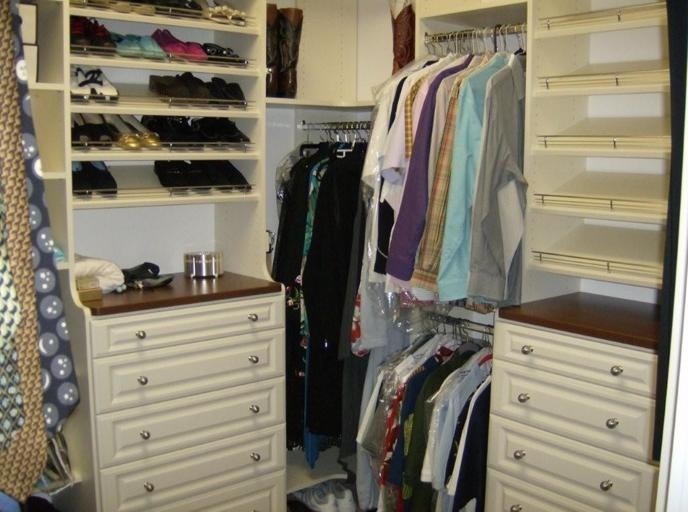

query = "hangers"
[372,308,494,406]
[386,23,525,75]
[275,119,375,167]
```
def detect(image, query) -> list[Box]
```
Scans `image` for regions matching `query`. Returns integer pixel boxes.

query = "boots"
[281,8,303,100]
[266,0,280,101]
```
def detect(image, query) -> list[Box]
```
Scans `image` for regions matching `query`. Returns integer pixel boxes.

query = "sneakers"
[67,64,120,103]
[0,4,24,455]
[293,481,356,511]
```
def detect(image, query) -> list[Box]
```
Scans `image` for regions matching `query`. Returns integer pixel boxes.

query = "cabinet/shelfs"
[263,1,393,510]
[393,3,528,512]
[9,0,287,512]
[484,1,671,512]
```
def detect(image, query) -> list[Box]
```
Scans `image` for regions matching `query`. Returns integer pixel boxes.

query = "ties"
[1,1,48,505]
[17,23,80,444]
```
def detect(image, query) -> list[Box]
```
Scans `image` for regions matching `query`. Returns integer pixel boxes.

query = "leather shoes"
[118,115,162,148]
[126,33,166,59]
[148,70,210,106]
[101,112,142,151]
[191,114,251,145]
[210,156,231,190]
[72,160,91,195]
[216,158,250,189]
[152,27,191,59]
[163,28,208,62]
[110,26,143,58]
[87,17,117,51]
[70,16,92,52]
[204,75,246,105]
[88,162,117,194]
[142,114,206,149]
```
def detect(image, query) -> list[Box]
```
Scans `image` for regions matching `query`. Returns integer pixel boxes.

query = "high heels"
[71,112,91,148]
[81,111,118,147]
[195,0,247,26]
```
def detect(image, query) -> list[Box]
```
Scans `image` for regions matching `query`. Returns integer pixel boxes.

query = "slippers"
[154,160,192,194]
[186,158,215,190]
